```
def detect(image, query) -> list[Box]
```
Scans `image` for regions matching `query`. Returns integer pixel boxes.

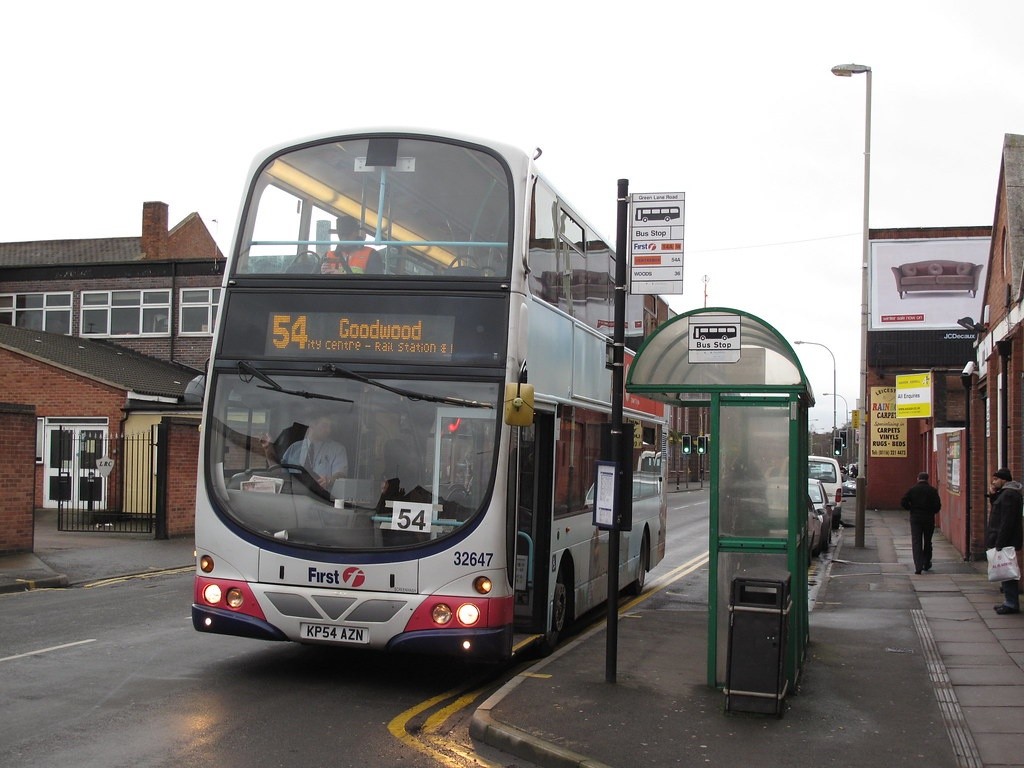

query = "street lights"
[794,341,837,436]
[831,64,873,550]
[823,393,848,427]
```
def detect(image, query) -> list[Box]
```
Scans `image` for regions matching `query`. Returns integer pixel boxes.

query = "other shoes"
[915,571,921,575]
[993,603,1020,615]
[923,561,932,571]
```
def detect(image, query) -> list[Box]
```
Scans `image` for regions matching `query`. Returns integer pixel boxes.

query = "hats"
[993,467,1012,482]
[328,215,363,236]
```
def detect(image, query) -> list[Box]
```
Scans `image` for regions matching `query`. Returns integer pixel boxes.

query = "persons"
[840,464,858,478]
[321,215,384,274]
[257,410,348,493]
[985,468,1023,614]
[375,438,415,513]
[902,472,941,574]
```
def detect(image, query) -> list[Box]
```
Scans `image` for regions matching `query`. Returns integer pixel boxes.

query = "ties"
[302,442,314,473]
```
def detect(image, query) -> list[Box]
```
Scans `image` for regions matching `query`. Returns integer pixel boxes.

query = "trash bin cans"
[722,566,792,721]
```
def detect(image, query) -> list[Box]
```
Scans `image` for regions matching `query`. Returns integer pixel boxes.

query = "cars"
[765,476,838,566]
[583,474,661,508]
[814,471,856,497]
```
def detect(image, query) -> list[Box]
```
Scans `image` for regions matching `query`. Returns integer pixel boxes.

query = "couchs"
[890,259,984,299]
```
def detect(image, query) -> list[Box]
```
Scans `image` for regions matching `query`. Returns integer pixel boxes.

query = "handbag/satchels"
[986,546,1020,583]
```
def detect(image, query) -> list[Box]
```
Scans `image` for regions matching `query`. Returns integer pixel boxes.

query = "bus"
[183,126,669,668]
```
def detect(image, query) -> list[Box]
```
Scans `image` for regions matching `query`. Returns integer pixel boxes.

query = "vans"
[808,455,847,529]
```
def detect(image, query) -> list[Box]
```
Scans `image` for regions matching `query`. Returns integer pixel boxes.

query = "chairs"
[445,253,480,276]
[284,250,320,274]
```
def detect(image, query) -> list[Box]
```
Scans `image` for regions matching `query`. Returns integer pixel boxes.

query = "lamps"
[957,317,987,335]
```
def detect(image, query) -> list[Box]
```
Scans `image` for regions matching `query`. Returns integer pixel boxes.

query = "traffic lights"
[682,434,693,455]
[697,436,707,455]
[834,437,842,456]
[839,431,847,448]
